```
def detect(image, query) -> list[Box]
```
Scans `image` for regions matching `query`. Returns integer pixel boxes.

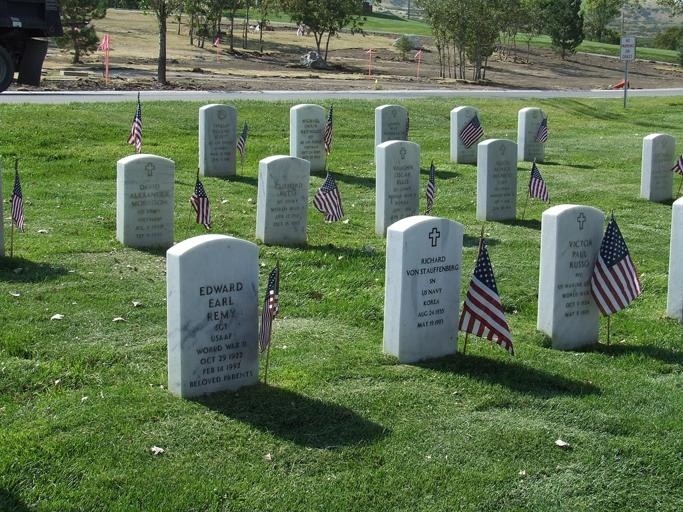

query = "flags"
[590,217,641,318]
[128,102,144,153]
[670,155,682,175]
[405,118,410,140]
[529,163,549,201]
[236,122,249,152]
[426,163,436,211]
[97,33,111,50]
[458,236,515,356]
[190,178,213,230]
[460,115,483,148]
[214,38,219,47]
[314,172,344,224]
[13,170,25,232]
[536,118,547,143]
[261,265,281,351]
[323,107,334,153]
